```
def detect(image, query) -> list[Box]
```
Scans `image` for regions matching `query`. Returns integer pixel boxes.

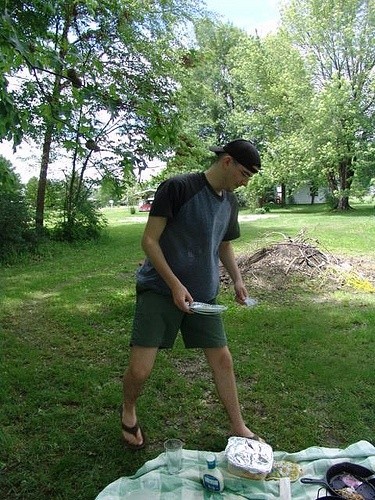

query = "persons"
[120,139,267,449]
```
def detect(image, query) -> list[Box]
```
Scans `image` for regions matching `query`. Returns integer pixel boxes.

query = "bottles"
[203,454,224,493]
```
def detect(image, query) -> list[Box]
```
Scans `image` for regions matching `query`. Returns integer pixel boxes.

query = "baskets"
[227,437,273,480]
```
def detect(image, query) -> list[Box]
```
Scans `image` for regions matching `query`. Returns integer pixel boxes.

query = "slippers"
[119,403,149,450]
[231,432,265,444]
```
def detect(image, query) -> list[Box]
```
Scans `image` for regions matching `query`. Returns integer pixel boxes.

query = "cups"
[164,439,185,474]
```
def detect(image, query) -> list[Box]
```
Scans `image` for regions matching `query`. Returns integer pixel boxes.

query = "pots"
[300,462,375,500]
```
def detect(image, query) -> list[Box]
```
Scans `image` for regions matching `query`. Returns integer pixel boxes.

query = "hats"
[209,139,261,174]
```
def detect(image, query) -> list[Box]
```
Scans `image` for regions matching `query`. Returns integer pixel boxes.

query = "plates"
[185,301,221,315]
[191,304,226,312]
